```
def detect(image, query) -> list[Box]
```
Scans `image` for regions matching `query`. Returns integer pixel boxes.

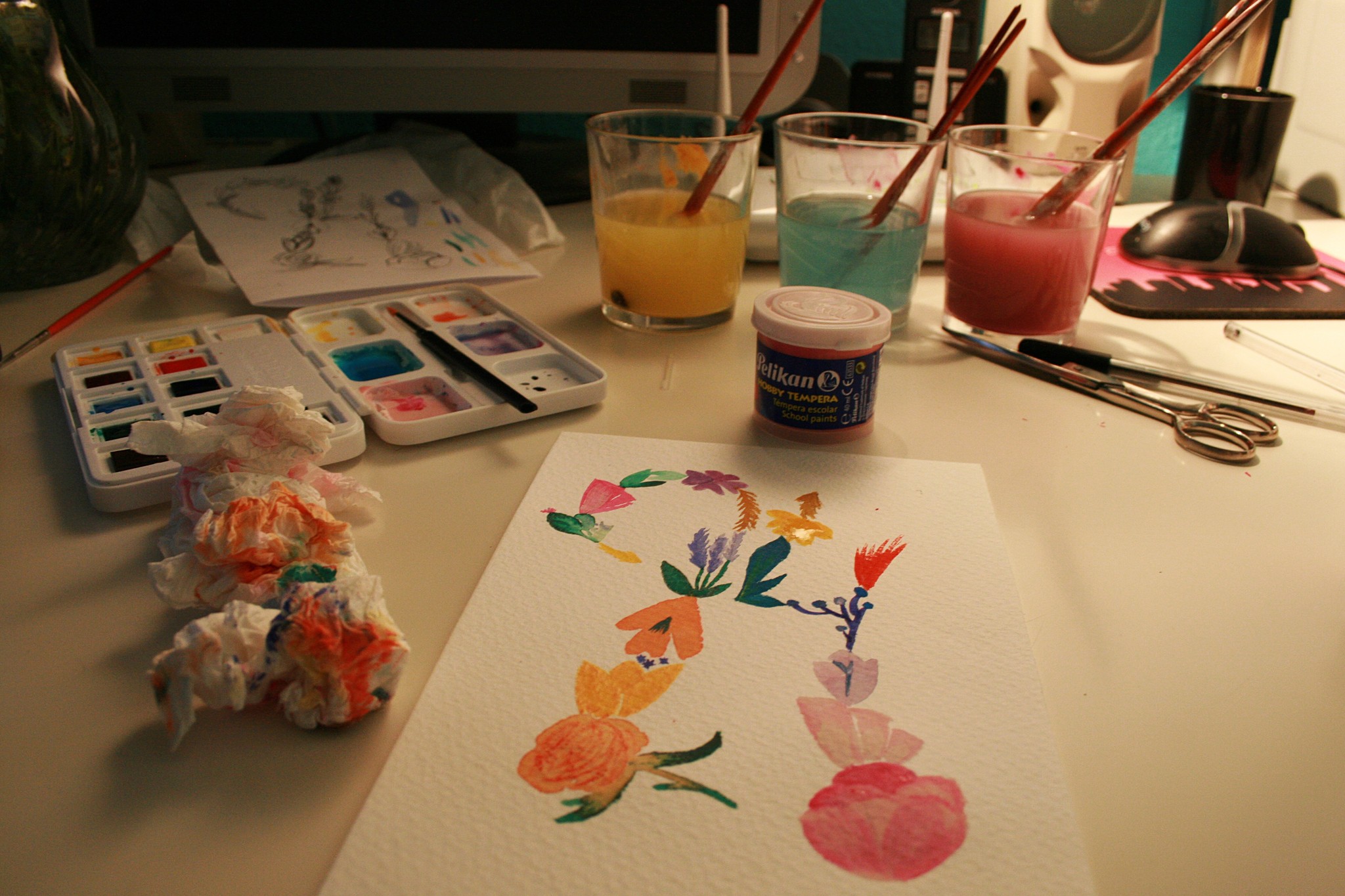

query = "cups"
[778,112,942,329]
[589,103,761,334]
[945,119,1129,345]
[1172,81,1295,214]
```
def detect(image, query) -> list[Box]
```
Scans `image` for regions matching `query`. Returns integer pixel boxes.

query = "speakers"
[977,1,1162,203]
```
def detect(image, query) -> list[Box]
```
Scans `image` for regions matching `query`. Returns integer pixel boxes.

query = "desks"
[3,174,1345,895]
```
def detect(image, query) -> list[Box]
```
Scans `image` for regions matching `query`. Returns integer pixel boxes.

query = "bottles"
[4,0,144,291]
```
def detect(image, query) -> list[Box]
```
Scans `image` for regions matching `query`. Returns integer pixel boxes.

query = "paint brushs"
[864,3,1023,220]
[1,234,173,369]
[867,19,1030,227]
[385,304,539,414]
[1035,0,1276,220]
[1027,0,1257,230]
[683,0,825,215]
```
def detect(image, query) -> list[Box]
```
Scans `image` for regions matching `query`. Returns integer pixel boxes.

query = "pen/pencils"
[1015,334,1345,433]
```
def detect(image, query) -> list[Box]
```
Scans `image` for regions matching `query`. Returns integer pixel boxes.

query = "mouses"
[1117,199,1320,279]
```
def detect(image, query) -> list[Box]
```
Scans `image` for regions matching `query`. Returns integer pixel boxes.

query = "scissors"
[940,321,1282,466]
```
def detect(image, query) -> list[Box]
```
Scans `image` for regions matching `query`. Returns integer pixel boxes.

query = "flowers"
[508,456,974,883]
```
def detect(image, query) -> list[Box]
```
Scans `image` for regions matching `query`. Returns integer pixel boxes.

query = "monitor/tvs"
[69,0,820,117]
